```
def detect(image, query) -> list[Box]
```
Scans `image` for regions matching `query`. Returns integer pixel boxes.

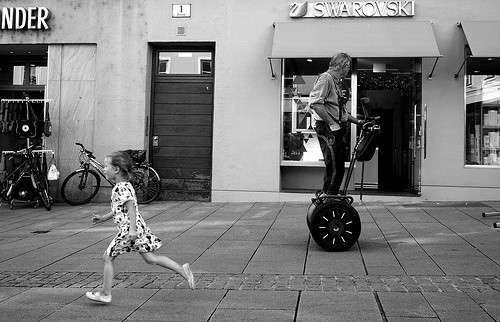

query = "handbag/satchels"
[357,123,381,161]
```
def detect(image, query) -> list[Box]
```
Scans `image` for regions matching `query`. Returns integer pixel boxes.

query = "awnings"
[454,20,500,78]
[269,21,442,80]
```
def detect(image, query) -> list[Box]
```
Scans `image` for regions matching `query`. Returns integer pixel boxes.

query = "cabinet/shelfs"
[465,101,500,166]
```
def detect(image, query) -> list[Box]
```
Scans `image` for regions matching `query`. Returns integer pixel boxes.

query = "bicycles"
[0,140,54,210]
[60,142,161,206]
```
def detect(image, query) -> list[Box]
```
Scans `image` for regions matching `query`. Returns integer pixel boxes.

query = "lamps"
[373,62,387,74]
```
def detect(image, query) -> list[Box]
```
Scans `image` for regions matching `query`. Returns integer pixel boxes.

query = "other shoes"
[86,292,112,302]
[182,263,194,290]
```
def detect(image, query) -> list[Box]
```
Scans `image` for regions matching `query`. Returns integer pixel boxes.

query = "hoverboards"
[306,114,382,251]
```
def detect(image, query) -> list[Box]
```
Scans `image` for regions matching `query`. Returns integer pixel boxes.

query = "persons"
[85,151,194,303]
[309,52,364,203]
